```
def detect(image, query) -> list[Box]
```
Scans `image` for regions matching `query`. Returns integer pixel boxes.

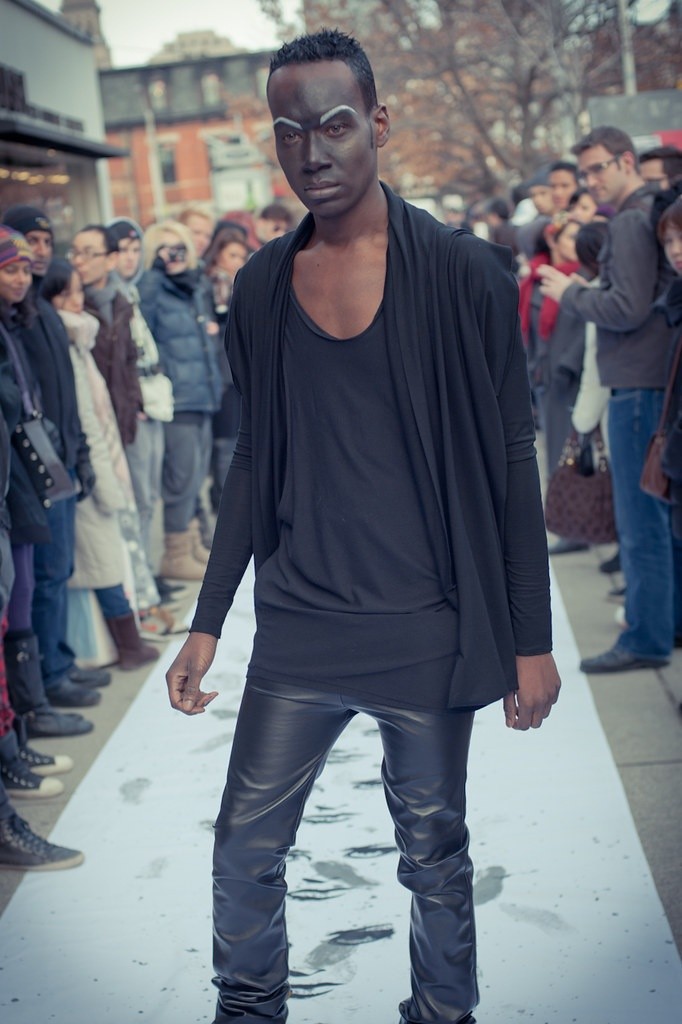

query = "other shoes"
[548,536,588,554]
[139,574,189,633]
[580,643,665,674]
[46,662,111,707]
[25,710,93,739]
[601,551,628,627]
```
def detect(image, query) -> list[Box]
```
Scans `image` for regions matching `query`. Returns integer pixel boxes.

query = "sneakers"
[1,758,64,798]
[0,812,84,870]
[2,745,73,775]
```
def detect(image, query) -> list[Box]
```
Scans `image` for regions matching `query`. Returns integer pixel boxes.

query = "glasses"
[64,245,109,261]
[576,156,616,187]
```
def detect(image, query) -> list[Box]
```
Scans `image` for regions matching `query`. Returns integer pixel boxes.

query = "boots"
[161,516,211,582]
[107,608,159,671]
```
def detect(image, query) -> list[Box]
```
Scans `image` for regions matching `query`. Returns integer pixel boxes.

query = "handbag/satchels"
[638,427,673,506]
[11,410,76,509]
[545,423,618,543]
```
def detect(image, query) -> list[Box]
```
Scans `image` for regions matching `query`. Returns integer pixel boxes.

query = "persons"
[0,204,300,871]
[166,29,561,1024]
[442,127,682,720]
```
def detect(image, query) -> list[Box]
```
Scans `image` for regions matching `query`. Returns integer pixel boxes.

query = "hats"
[512,187,530,206]
[442,195,462,210]
[5,205,54,237]
[526,169,549,190]
[110,222,141,241]
[493,201,508,220]
[0,224,36,271]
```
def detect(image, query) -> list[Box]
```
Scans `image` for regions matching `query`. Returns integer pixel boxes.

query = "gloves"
[77,461,96,502]
